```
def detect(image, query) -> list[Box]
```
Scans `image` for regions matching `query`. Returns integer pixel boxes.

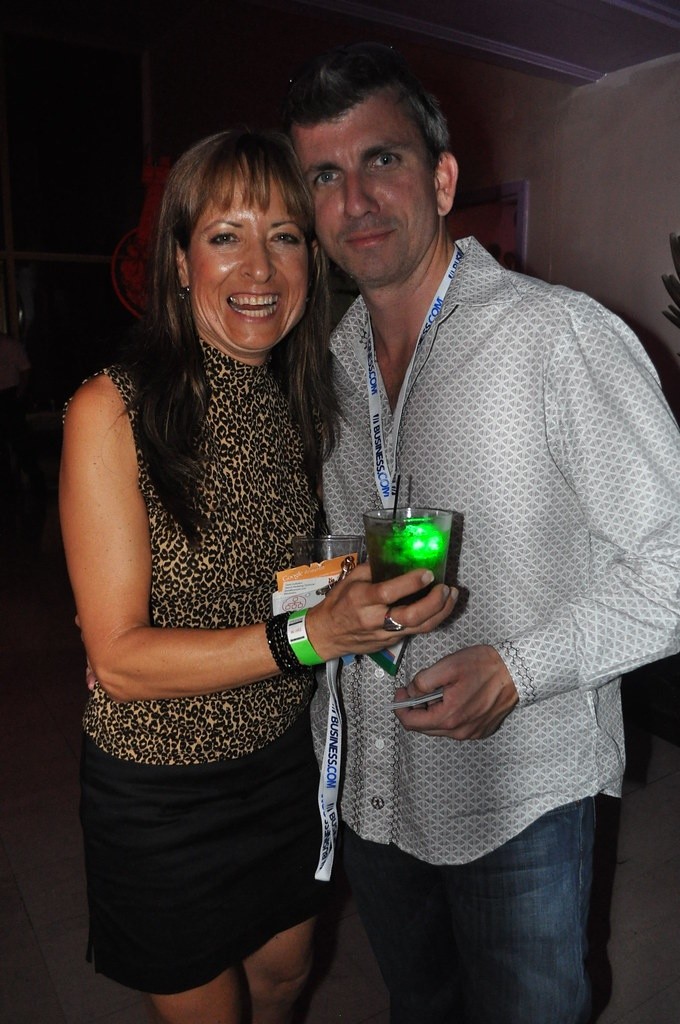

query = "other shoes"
[34,476,45,495]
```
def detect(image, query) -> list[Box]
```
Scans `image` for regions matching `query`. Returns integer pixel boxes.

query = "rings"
[382,607,408,632]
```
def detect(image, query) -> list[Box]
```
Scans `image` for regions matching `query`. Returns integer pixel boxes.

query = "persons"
[56,40,680,1024]
[0,331,46,497]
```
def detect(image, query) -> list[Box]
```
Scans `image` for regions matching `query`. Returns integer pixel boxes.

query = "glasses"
[284,42,436,121]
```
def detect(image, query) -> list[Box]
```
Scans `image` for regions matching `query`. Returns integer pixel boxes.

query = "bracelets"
[264,608,326,676]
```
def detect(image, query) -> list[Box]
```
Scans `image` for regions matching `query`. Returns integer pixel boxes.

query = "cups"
[292,535,361,572]
[363,508,452,606]
